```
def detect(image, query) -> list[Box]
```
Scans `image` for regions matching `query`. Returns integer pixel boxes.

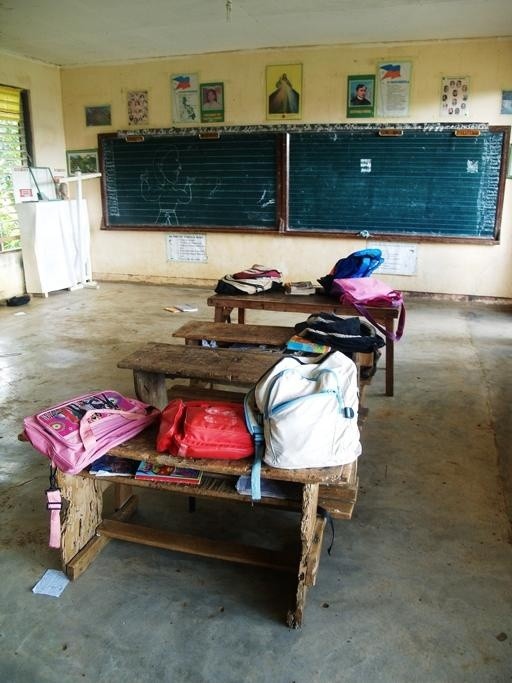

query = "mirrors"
[29,165,63,199]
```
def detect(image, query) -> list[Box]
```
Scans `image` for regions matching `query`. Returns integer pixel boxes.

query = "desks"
[209,275,399,399]
[16,319,376,631]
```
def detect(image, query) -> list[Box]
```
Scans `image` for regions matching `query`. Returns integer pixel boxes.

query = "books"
[235,475,297,501]
[283,280,316,296]
[88,454,203,486]
[164,303,199,313]
[286,334,331,354]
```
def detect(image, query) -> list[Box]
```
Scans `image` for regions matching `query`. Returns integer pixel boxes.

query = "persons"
[443,80,468,115]
[350,84,372,105]
[203,89,222,108]
[269,73,299,114]
[128,94,148,124]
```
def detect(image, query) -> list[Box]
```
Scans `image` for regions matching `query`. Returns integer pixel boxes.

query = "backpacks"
[214,264,284,296]
[316,249,385,290]
[243,349,362,501]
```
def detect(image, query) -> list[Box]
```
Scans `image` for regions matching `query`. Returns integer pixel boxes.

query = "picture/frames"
[65,148,101,176]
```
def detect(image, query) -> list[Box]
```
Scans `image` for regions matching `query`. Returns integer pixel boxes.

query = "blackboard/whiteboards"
[97,124,511,245]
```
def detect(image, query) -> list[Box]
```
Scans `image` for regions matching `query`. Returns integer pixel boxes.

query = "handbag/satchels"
[23,390,162,550]
[295,311,385,355]
[156,397,253,460]
[329,276,406,343]
